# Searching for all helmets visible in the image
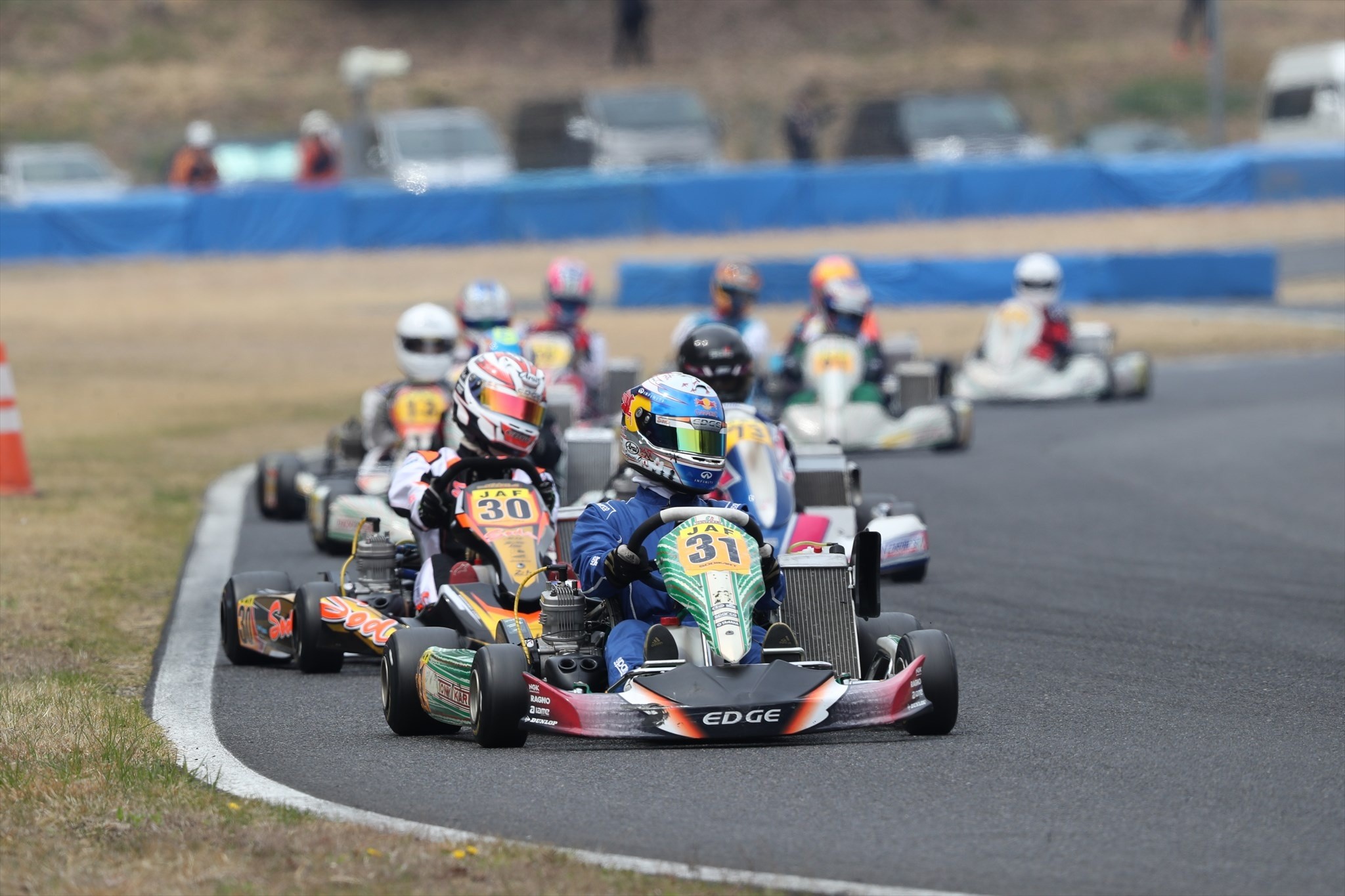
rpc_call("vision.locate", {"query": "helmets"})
[708,259,760,318]
[540,255,594,325]
[807,255,873,338]
[394,303,460,384]
[461,280,513,347]
[1011,251,1062,310]
[451,351,547,455]
[677,325,754,402]
[620,370,729,495]
[799,331,866,397]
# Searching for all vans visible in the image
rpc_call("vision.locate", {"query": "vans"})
[1262,46,1343,149]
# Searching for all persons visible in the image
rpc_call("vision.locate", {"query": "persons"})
[977,251,1070,363]
[569,371,800,693]
[298,109,343,181]
[1175,0,1223,52]
[361,251,887,512]
[169,121,219,188]
[387,351,576,615]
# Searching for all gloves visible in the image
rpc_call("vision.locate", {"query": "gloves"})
[418,485,456,528]
[759,542,781,585]
[532,479,556,513]
[606,543,653,588]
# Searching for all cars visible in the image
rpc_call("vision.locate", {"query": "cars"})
[508,84,727,173]
[787,83,1060,160]
[358,105,517,184]
[3,142,146,201]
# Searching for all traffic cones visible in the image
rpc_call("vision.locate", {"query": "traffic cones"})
[0,338,39,498]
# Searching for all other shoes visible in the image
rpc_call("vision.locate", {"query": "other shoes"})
[549,561,578,582]
[640,624,679,675]
[449,561,479,584]
[760,622,801,663]
